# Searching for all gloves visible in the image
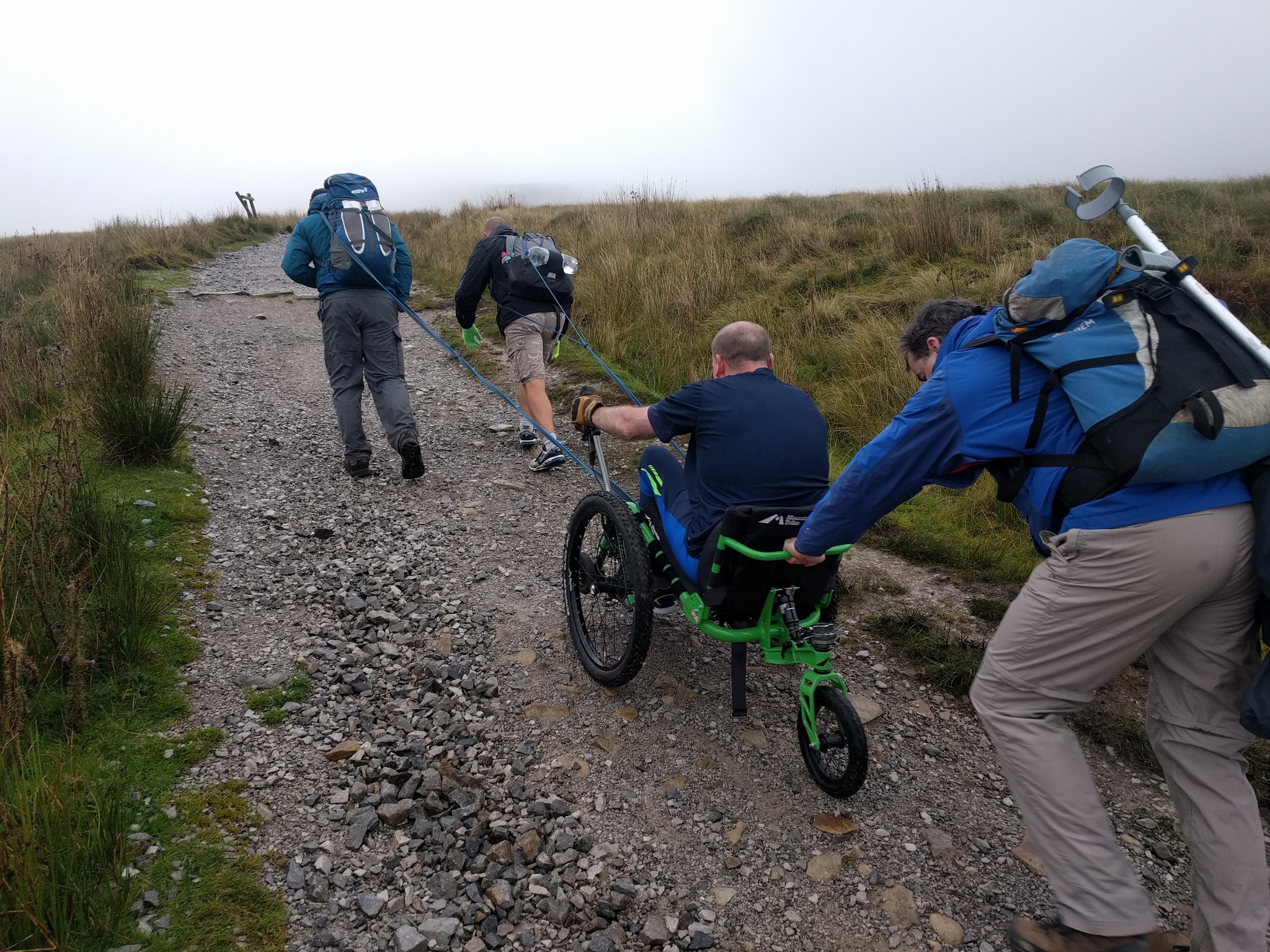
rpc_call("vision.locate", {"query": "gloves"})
[462,324,483,349]
[551,340,560,360]
[570,395,605,434]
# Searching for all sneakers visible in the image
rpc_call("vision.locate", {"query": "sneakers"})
[518,429,539,446]
[529,445,566,472]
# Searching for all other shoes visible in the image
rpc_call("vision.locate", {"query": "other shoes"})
[343,457,370,476]
[652,586,677,615]
[398,429,425,479]
[1012,915,1149,952]
[1160,929,1190,952]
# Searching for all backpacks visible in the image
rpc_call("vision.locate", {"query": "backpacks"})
[958,237,1270,486]
[494,234,574,305]
[323,172,396,286]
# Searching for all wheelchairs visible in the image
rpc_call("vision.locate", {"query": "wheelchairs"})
[554,386,871,799]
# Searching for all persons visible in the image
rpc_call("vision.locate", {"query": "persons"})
[783,301,1270,952]
[281,188,427,478]
[454,217,573,471]
[571,321,831,615]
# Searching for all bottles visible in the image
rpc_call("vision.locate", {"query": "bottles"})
[501,252,520,282]
[523,246,578,275]
